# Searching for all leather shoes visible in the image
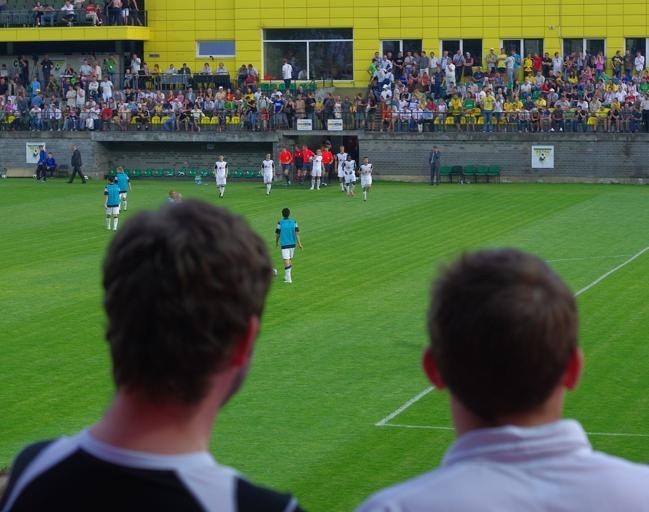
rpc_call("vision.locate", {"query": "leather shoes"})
[66,181,73,183]
[82,180,86,184]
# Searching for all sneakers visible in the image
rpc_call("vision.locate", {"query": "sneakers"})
[317,188,320,190]
[219,196,224,198]
[364,199,366,201]
[322,183,327,186]
[351,194,354,197]
[348,195,350,196]
[113,228,117,231]
[284,280,292,283]
[342,190,346,192]
[310,188,314,190]
[266,194,270,196]
[106,227,110,230]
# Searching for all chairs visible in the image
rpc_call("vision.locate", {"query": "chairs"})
[260,81,318,92]
[463,166,476,183]
[0,0,106,26]
[449,166,462,183]
[105,164,268,179]
[475,165,487,184]
[130,114,244,126]
[432,113,507,127]
[438,166,449,183]
[487,164,501,183]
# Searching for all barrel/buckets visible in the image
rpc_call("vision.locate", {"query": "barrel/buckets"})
[194,175,202,184]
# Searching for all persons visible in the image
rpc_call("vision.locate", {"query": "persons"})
[260,140,373,202]
[351,247,649,512]
[104,177,121,230]
[35,143,56,182]
[68,145,86,184]
[1,53,367,130]
[114,166,131,211]
[275,208,302,283]
[1,197,306,512]
[33,0,143,26]
[367,48,649,133]
[215,154,228,197]
[429,146,440,185]
